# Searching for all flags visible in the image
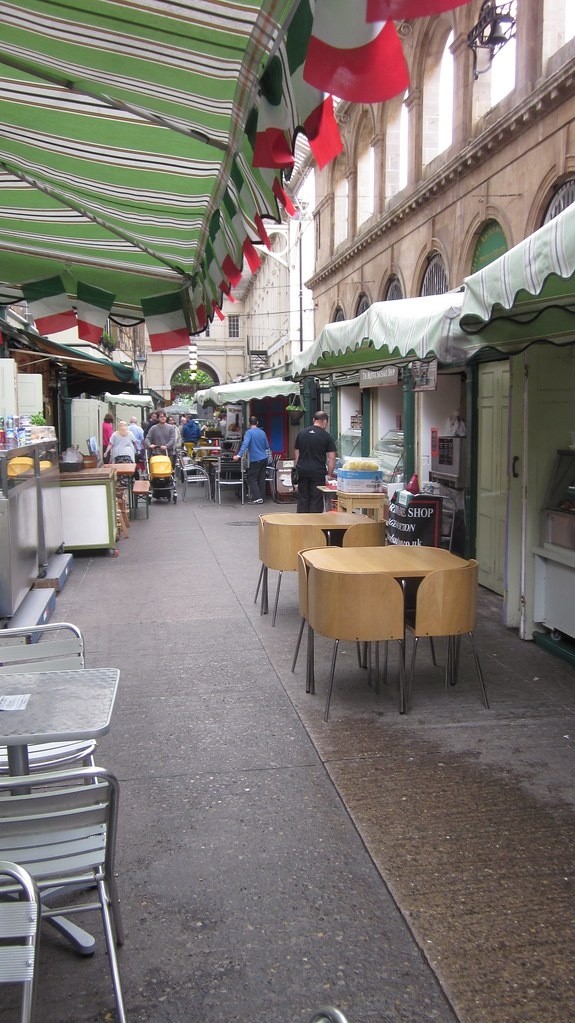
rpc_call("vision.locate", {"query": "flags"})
[19,0,468,350]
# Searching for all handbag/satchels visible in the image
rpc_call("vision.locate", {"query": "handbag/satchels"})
[291,468,299,486]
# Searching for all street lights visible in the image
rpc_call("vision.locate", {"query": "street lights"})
[136,353,147,422]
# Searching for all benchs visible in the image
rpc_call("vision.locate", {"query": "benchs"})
[132,480,151,520]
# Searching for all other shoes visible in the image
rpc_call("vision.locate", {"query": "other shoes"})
[253,498,264,503]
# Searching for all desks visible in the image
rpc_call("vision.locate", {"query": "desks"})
[202,456,235,502]
[194,446,221,457]
[305,546,467,695]
[263,513,380,615]
[104,462,138,519]
[318,486,337,513]
[0,667,122,955]
[338,492,385,523]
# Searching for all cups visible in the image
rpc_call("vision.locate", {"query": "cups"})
[17,428,25,448]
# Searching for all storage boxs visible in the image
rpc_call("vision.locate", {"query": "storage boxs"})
[335,469,383,491]
[83,457,97,469]
[342,456,378,472]
[59,463,81,472]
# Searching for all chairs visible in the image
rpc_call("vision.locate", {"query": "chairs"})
[0,621,128,1023]
[214,457,243,505]
[252,511,490,722]
[178,450,212,502]
[245,453,277,500]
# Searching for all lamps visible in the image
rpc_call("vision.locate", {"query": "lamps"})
[189,351,197,358]
[188,342,197,351]
[188,358,197,365]
[190,370,197,376]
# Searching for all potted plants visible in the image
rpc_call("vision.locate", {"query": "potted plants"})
[100,330,116,352]
[286,406,308,425]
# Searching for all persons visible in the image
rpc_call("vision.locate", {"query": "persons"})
[104,421,139,463]
[141,411,183,477]
[128,416,145,479]
[103,414,113,463]
[232,416,269,504]
[180,415,208,448]
[294,411,336,512]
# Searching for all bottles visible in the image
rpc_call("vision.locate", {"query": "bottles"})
[5,415,15,450]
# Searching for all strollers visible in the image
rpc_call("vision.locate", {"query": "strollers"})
[145,445,177,504]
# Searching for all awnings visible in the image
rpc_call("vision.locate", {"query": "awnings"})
[193,379,301,409]
[292,293,574,378]
[139,389,166,403]
[0,0,301,336]
[7,328,140,384]
[104,393,153,409]
[458,201,574,336]
